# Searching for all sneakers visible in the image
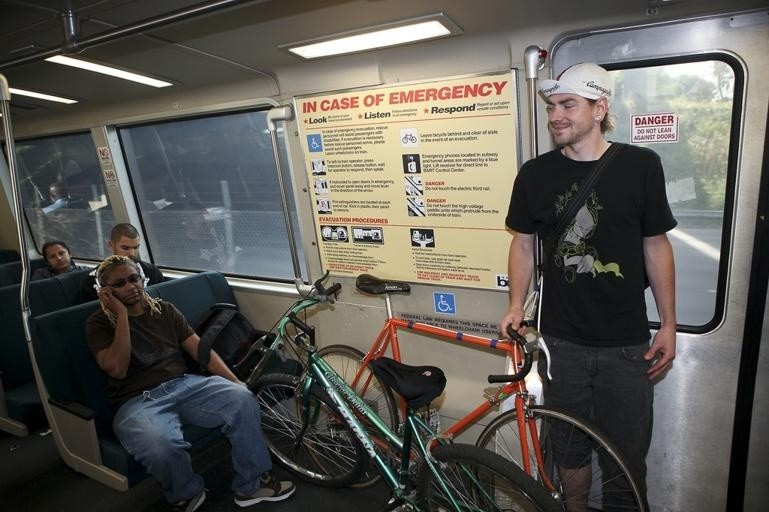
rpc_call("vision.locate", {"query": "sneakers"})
[167,483,208,512]
[231,471,296,508]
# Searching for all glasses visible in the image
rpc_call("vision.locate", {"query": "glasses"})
[98,271,142,290]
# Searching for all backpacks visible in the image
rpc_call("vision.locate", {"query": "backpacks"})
[190,302,266,380]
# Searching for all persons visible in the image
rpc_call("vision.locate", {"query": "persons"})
[85,254,296,511]
[503,62,678,510]
[31,241,81,281]
[77,223,166,304]
[40,182,71,214]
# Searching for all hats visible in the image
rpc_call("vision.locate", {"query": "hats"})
[537,60,615,110]
